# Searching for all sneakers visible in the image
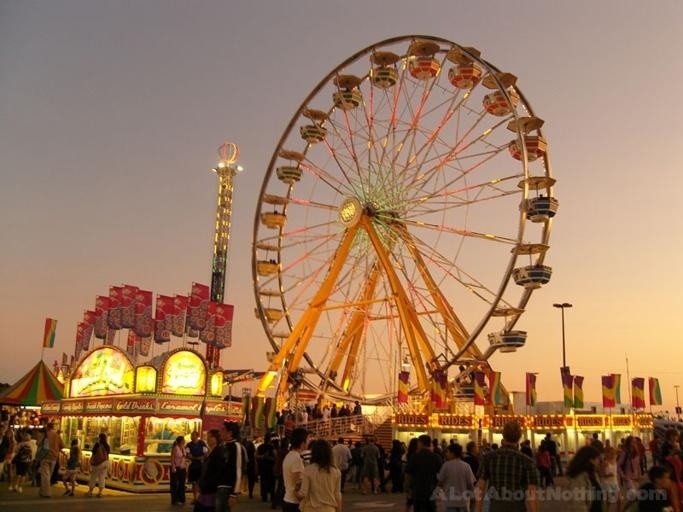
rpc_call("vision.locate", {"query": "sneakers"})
[41,496,51,498]
[96,494,102,497]
[85,493,92,497]
[374,488,381,495]
[69,493,74,496]
[362,490,368,495]
[64,490,70,495]
[176,502,183,505]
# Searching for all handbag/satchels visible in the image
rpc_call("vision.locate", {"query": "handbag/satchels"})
[170,472,183,483]
[90,441,108,466]
[35,432,49,461]
[12,445,26,465]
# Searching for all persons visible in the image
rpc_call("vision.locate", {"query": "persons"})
[1,370,682,512]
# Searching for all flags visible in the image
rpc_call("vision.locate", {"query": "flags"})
[43,281,234,368]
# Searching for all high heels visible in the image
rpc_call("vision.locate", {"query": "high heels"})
[8,485,24,496]
